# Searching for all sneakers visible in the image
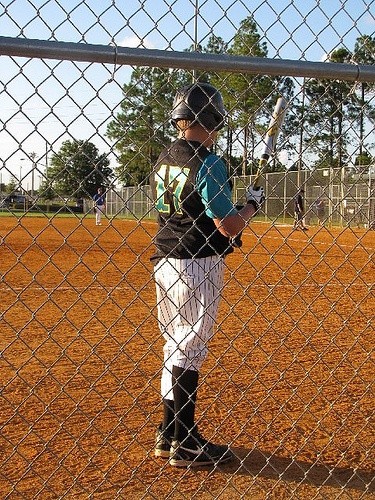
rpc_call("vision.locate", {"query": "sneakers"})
[153,423,174,458]
[292,227,299,231]
[170,438,233,466]
[302,227,309,231]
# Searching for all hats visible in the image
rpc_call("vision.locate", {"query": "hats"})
[299,189,306,193]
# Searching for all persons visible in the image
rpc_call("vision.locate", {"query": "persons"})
[292,189,309,231]
[93,188,106,226]
[315,196,325,226]
[153,83,287,466]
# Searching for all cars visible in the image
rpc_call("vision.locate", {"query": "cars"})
[75,200,83,211]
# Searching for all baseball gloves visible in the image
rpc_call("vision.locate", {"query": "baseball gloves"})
[92,207,96,213]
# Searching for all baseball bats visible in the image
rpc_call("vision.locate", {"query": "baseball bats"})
[230,94,287,245]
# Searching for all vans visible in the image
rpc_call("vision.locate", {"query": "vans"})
[2,195,29,205]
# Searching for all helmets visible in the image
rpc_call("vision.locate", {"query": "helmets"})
[171,82,225,131]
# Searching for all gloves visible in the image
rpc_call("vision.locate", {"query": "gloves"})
[245,183,265,213]
